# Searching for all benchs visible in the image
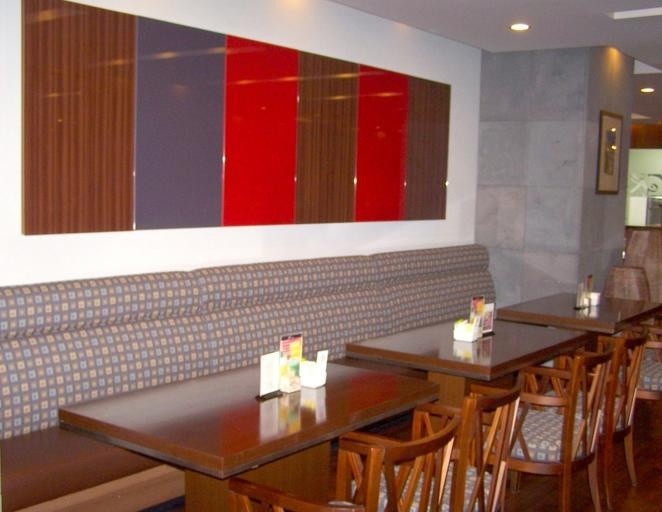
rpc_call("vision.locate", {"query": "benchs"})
[0,245,499,512]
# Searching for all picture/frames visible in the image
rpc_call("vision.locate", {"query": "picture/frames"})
[596,113,624,195]
